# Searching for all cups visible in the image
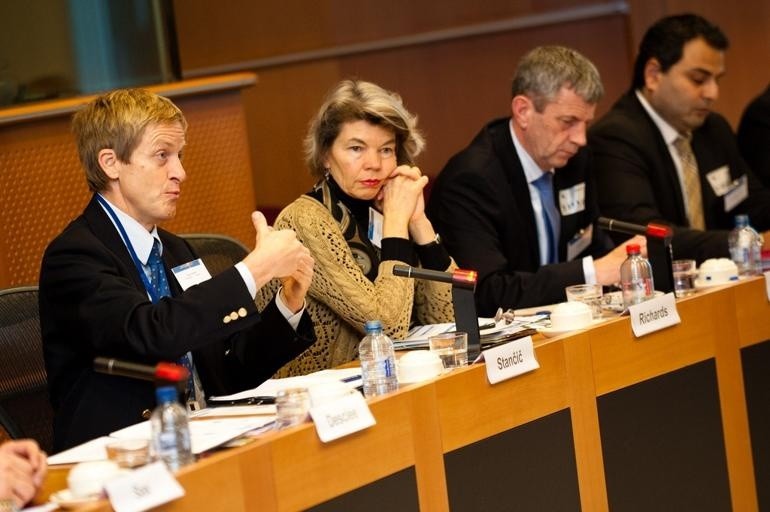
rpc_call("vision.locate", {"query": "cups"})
[68,461,104,495]
[671,259,696,296]
[428,331,469,370]
[551,301,592,328]
[697,258,737,283]
[276,387,311,427]
[567,283,602,321]
[107,440,149,469]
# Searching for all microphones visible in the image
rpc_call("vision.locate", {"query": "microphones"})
[393,264,480,291]
[596,216,675,249]
[92,356,189,392]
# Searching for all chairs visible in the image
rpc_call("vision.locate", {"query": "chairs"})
[1,284,56,456]
[177,231,251,277]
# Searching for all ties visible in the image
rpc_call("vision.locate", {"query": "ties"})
[149,238,195,399]
[532,171,561,265]
[674,131,706,232]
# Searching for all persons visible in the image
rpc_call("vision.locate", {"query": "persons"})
[255,80,460,379]
[586,12,770,269]
[429,45,648,319]
[0,438,48,510]
[737,84,770,187]
[38,89,317,455]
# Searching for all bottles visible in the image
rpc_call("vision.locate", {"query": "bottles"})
[359,321,398,396]
[730,216,762,279]
[153,388,190,467]
[620,244,654,310]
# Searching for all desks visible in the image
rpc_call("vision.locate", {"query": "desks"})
[5,256,764,511]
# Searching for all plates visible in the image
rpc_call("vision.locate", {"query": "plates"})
[48,489,95,509]
[399,350,444,377]
[693,283,732,291]
[396,378,425,387]
[538,329,573,338]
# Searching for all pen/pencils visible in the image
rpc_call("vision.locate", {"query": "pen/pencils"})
[344,375,362,382]
[479,323,495,330]
